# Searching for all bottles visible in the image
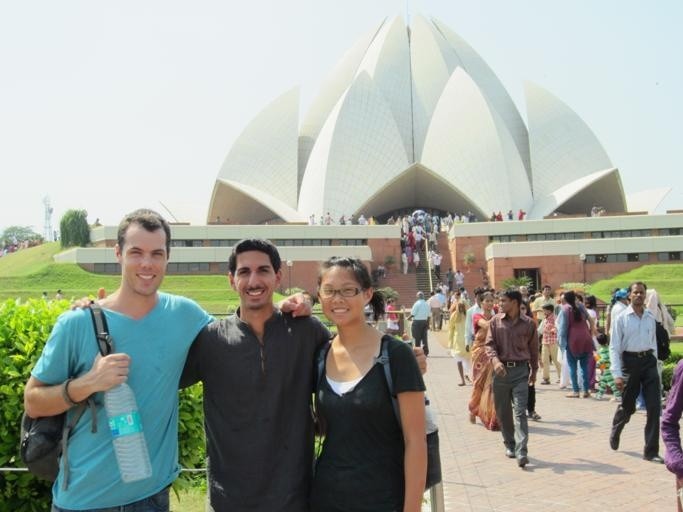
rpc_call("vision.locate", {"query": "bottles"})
[424,397,436,435]
[103,381,152,482]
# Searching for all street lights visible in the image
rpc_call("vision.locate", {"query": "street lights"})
[578,253,589,294]
[286,260,296,294]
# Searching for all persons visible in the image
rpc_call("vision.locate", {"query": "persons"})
[637,288,675,410]
[482,288,539,468]
[364,263,500,430]
[661,358,683,511]
[0,237,42,256]
[213,215,231,225]
[587,205,607,217]
[519,284,629,397]
[24,208,313,512]
[94,217,101,226]
[608,282,664,464]
[310,253,429,511]
[461,209,526,222]
[309,212,376,224]
[66,238,428,512]
[389,213,465,279]
[42,289,64,301]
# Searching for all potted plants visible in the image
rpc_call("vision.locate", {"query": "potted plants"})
[461,253,475,273]
[382,256,397,274]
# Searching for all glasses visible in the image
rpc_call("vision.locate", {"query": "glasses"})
[318,288,362,298]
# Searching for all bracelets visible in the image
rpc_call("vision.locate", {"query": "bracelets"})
[61,378,81,409]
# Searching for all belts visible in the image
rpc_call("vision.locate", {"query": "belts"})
[503,361,527,368]
[623,349,652,358]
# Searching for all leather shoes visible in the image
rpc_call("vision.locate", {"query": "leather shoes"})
[648,456,663,464]
[505,448,528,468]
[609,435,618,450]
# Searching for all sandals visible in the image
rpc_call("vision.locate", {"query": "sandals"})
[528,411,540,419]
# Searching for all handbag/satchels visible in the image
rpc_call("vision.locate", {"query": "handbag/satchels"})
[655,321,669,360]
[20,410,65,481]
